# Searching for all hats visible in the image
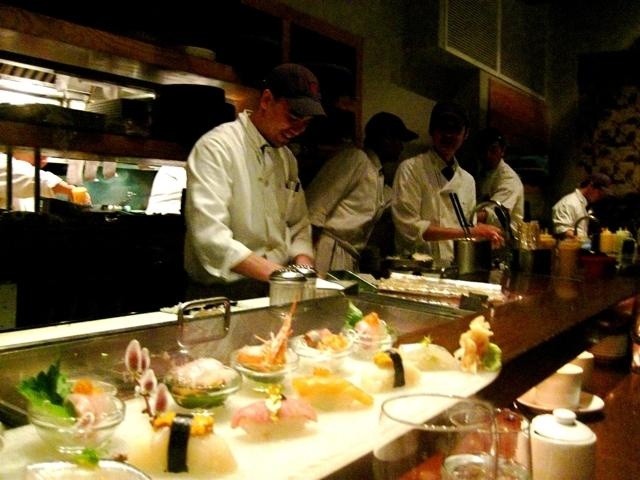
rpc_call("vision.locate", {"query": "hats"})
[430,100,467,125]
[266,63,326,116]
[364,111,419,143]
[587,174,614,196]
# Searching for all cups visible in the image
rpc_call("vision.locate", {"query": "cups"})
[442,408,530,480]
[453,238,492,276]
[571,349,594,384]
[537,363,581,410]
[372,395,499,480]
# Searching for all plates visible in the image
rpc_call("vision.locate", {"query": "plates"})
[1,366,500,480]
[590,335,627,358]
[518,384,604,416]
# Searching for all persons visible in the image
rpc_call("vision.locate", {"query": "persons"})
[475,129,526,232]
[1,149,92,214]
[144,165,188,216]
[305,110,420,281]
[391,96,501,268]
[183,62,315,298]
[551,176,606,239]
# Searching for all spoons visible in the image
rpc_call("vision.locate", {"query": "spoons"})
[494,206,519,250]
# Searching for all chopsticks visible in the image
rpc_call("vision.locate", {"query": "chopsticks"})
[449,192,473,242]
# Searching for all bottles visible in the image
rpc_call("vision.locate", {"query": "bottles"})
[517,409,597,480]
[599,227,631,253]
[267,268,305,320]
[286,263,320,310]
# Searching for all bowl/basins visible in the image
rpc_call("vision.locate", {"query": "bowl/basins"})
[344,325,398,360]
[164,366,246,410]
[229,344,299,394]
[25,395,123,457]
[51,378,117,404]
[21,456,153,480]
[287,330,356,362]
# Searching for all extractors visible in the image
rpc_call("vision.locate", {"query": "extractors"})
[1,62,73,98]
